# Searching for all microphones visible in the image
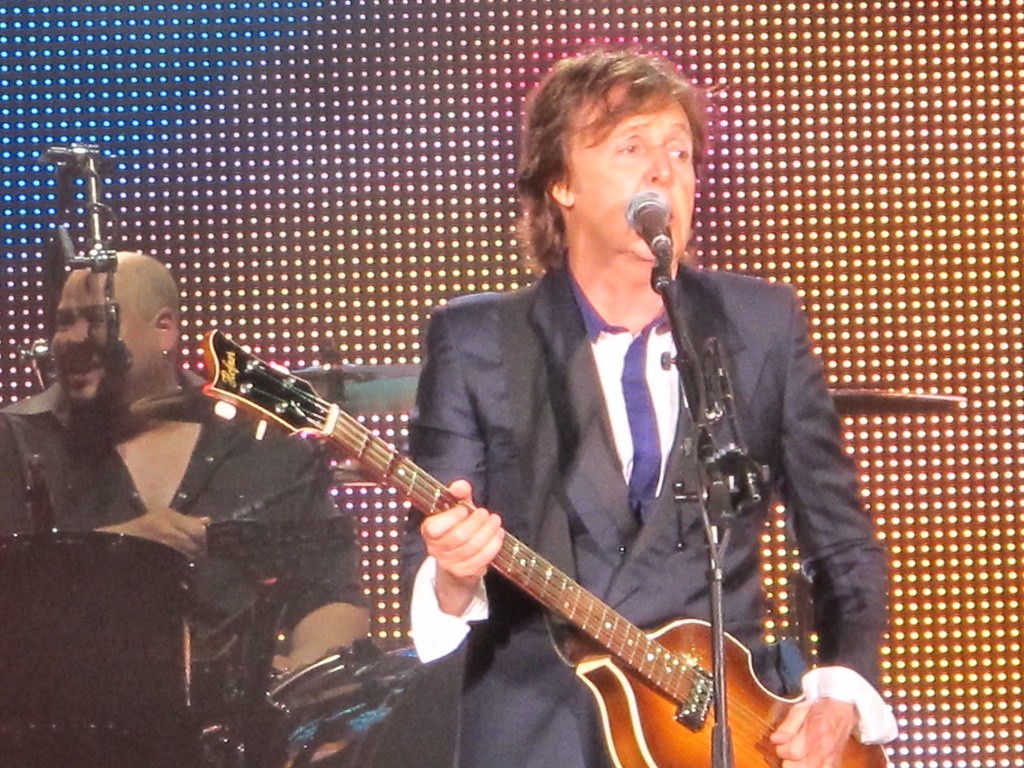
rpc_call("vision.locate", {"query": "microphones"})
[625,191,673,253]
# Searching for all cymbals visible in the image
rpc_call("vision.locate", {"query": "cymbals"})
[128,365,421,421]
[831,387,968,414]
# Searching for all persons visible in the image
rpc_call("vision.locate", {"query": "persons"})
[0,251,371,768]
[399,47,898,768]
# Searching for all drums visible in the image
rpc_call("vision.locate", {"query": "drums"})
[2,531,198,768]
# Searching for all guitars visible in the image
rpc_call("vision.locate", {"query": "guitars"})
[186,316,904,768]
